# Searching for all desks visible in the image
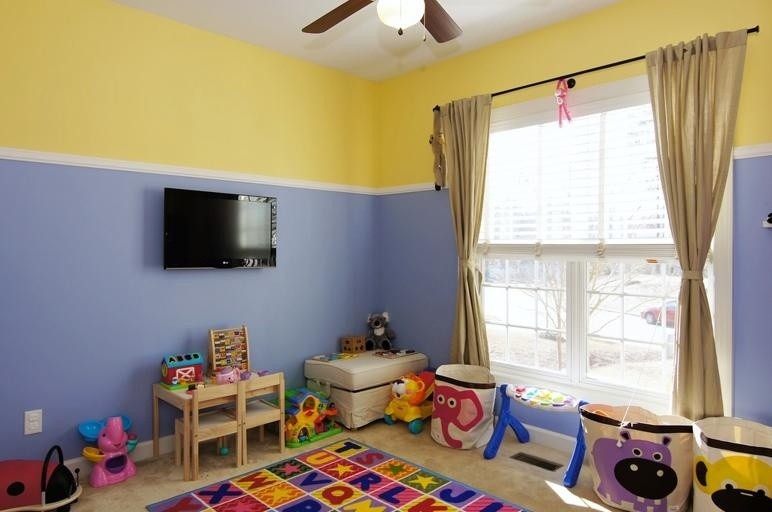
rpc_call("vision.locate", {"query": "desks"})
[151,369,286,482]
[303,347,429,433]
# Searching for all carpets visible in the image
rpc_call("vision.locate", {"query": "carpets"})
[143,436,537,511]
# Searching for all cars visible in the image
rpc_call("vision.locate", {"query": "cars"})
[637,298,678,329]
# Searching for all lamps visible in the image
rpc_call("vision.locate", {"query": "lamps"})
[375,0,430,43]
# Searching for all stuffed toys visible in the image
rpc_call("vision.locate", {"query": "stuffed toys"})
[554,80,572,128]
[366,311,396,352]
[429,106,446,192]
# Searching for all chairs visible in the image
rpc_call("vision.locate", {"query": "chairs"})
[221,370,285,466]
[173,381,244,481]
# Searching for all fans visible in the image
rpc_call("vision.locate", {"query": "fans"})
[300,1,461,46]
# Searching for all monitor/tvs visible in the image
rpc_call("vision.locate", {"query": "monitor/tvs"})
[163,188,276,270]
